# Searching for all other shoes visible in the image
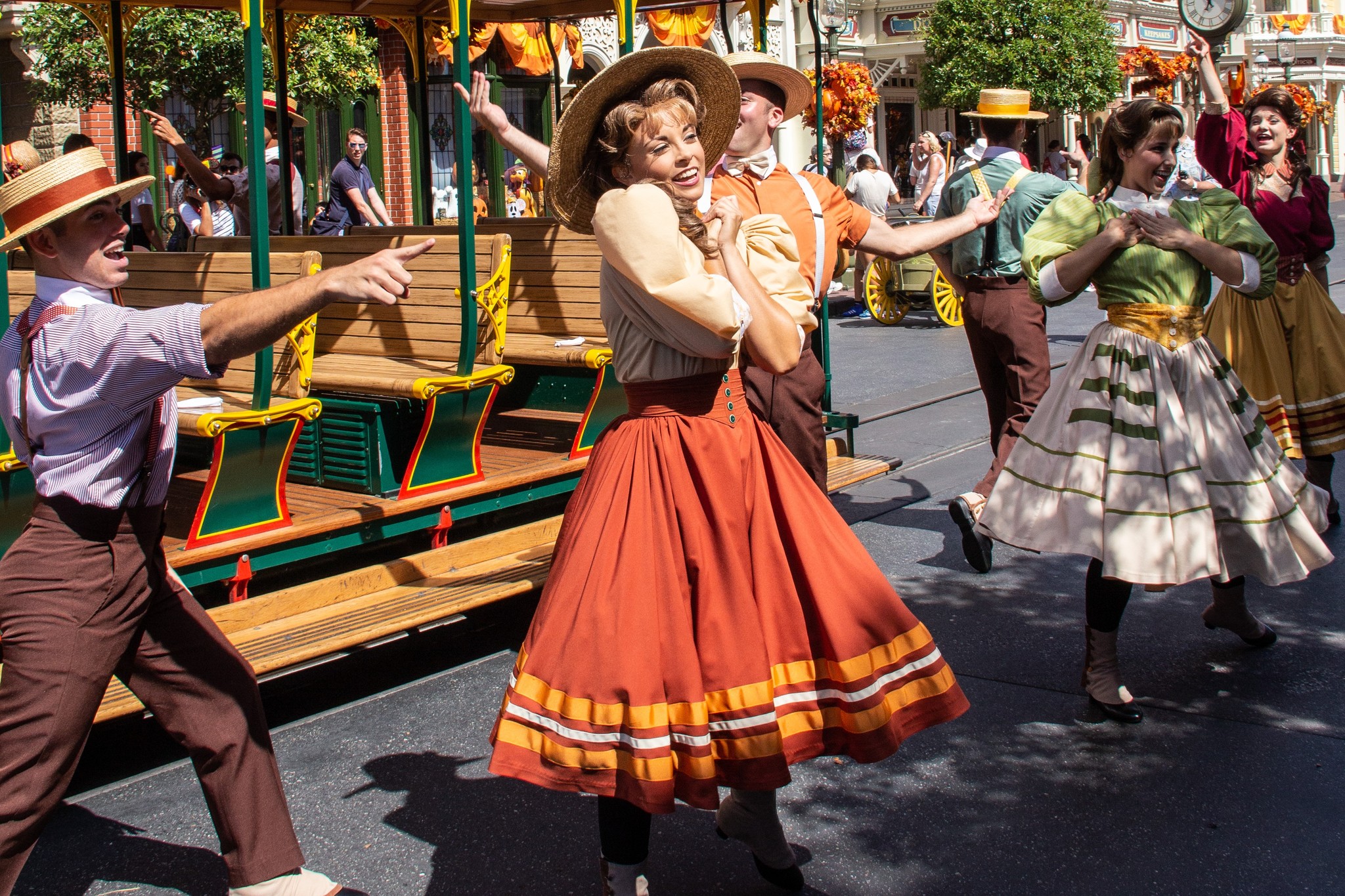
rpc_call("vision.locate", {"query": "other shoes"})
[949,492,994,573]
[226,872,369,896]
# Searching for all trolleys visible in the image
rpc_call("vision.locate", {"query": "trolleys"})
[862,208,964,327]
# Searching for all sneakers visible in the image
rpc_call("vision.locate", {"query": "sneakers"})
[842,306,864,317]
[859,308,885,319]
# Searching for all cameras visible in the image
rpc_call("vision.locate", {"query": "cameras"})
[184,174,199,189]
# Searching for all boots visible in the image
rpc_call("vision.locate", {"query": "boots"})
[716,790,805,891]
[1201,574,1277,647]
[1082,621,1143,724]
[598,848,672,896]
[1303,455,1341,526]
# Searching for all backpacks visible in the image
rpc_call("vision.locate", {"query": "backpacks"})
[1043,152,1060,175]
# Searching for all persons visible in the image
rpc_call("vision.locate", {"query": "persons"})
[1161,104,1222,202]
[143,90,310,236]
[167,149,244,252]
[1184,28,1345,525]
[843,115,884,184]
[921,88,1088,574]
[327,128,398,236]
[452,46,1009,504]
[1077,133,1095,178]
[801,143,843,294]
[840,154,901,319]
[125,151,166,252]
[909,129,988,218]
[973,96,1334,723]
[1043,140,1068,181]
[1,140,436,894]
[488,49,974,896]
[1,133,95,182]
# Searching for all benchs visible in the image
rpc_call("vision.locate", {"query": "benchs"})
[0,218,629,552]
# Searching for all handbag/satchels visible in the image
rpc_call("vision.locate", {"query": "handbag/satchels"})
[309,219,345,236]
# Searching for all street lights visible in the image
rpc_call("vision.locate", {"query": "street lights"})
[808,0,858,288]
[1271,23,1304,86]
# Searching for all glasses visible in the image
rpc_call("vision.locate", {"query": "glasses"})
[822,152,833,157]
[219,165,241,173]
[346,142,366,148]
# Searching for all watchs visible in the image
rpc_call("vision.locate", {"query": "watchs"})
[1191,181,1198,191]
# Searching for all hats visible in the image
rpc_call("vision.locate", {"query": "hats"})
[939,132,957,140]
[1,140,42,182]
[235,91,309,127]
[963,138,987,161]
[0,146,156,253]
[543,45,742,236]
[960,89,1050,120]
[721,51,813,123]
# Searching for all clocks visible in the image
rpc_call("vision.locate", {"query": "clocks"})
[1178,0,1248,36]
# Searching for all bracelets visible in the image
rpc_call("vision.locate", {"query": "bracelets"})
[387,221,394,226]
